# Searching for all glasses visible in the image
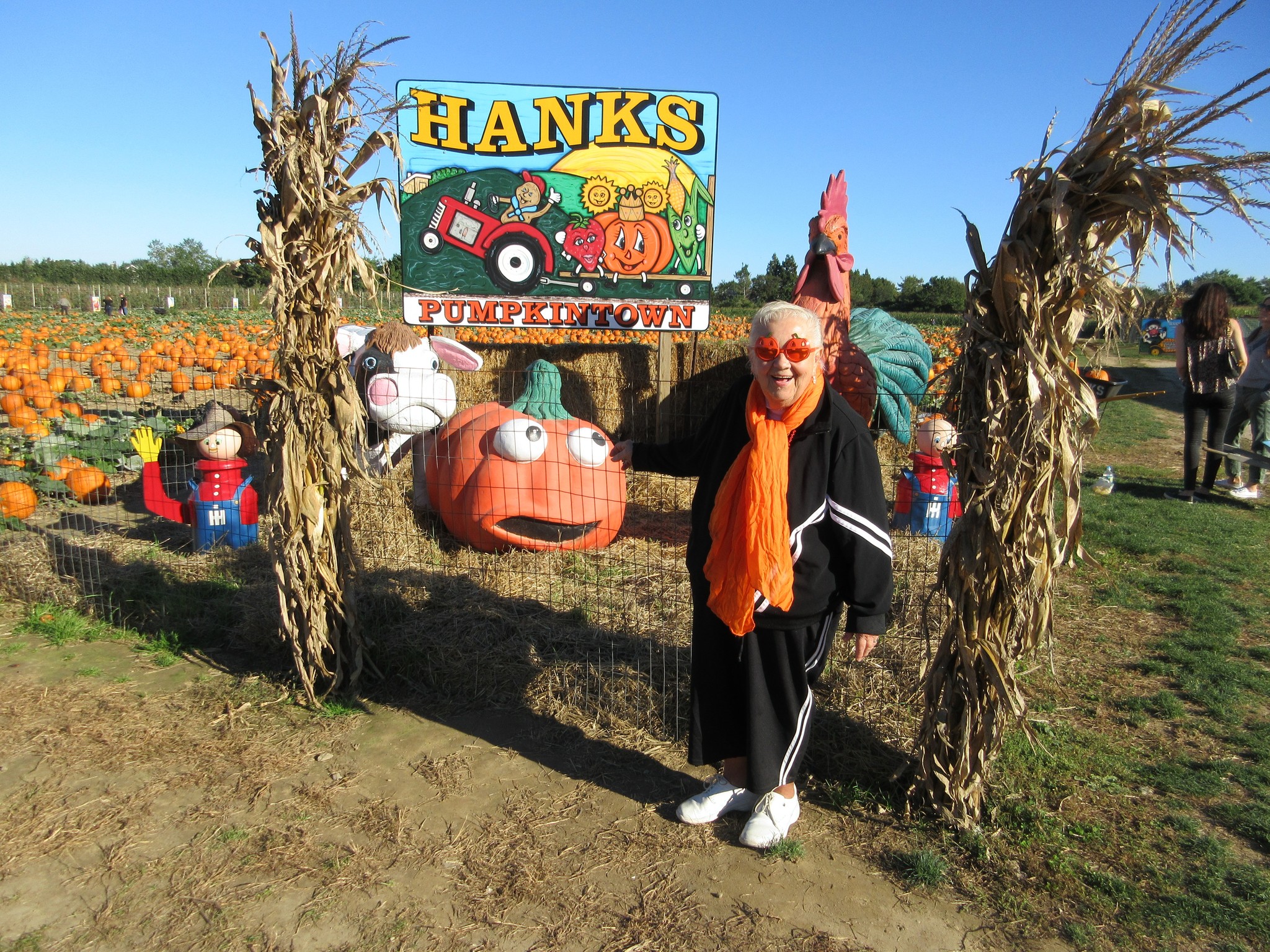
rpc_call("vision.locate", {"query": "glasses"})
[1259,303,1270,311]
[749,334,819,363]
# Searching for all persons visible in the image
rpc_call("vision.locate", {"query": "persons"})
[1163,283,1248,503]
[610,299,895,850]
[57,295,71,316]
[119,293,128,315]
[103,297,113,317]
[1208,296,1270,499]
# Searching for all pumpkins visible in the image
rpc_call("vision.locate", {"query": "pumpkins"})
[0,482,37,518]
[1086,369,1110,382]
[66,467,110,503]
[339,305,751,345]
[917,324,962,424]
[0,306,283,481]
[1068,362,1081,376]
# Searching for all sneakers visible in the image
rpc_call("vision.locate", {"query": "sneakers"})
[1179,489,1210,502]
[737,779,800,848]
[676,773,759,825]
[1226,487,1258,498]
[1214,479,1243,489]
[1163,493,1193,505]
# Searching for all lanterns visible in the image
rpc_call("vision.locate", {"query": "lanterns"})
[424,360,626,553]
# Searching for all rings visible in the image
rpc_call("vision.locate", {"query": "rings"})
[873,640,877,642]
